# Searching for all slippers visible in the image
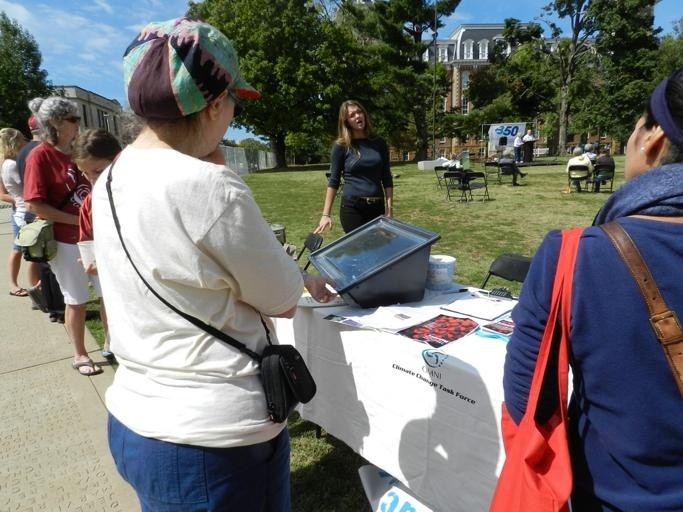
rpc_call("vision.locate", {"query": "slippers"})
[102,351,119,364]
[72,358,103,376]
[9,287,29,296]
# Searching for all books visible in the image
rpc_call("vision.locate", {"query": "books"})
[482,313,515,336]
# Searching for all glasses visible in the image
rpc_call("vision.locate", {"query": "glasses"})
[79,166,104,179]
[60,115,82,124]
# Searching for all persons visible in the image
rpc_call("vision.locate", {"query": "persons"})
[495,135,528,186]
[522,129,539,142]
[16,115,44,288]
[591,148,616,192]
[513,133,524,164]
[565,146,594,193]
[313,99,393,234]
[73,127,122,276]
[583,143,597,166]
[1,128,40,297]
[22,96,115,376]
[502,75,683,512]
[91,18,338,512]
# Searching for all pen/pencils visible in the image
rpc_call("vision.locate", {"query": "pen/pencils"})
[442,288,468,294]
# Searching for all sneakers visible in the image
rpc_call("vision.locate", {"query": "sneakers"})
[31,300,40,310]
[29,287,49,312]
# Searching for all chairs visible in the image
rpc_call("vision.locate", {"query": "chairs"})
[567,165,592,193]
[443,172,467,201]
[484,160,503,184]
[448,167,475,189]
[479,252,532,289]
[434,166,455,190]
[499,163,517,186]
[463,171,489,203]
[592,164,616,193]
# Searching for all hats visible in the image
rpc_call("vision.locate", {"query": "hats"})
[122,17,260,121]
[584,143,594,151]
[29,114,39,132]
[573,147,582,155]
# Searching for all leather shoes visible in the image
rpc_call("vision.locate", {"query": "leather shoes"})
[521,172,529,178]
[56,315,66,323]
[49,313,57,322]
[513,182,519,186]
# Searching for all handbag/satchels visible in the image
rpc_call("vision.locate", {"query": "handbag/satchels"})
[105,149,317,424]
[14,174,84,263]
[487,225,586,511]
[598,223,682,393]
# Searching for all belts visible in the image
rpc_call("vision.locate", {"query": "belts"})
[347,197,384,206]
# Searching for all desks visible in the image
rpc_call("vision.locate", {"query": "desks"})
[269,280,575,512]
[523,141,535,163]
